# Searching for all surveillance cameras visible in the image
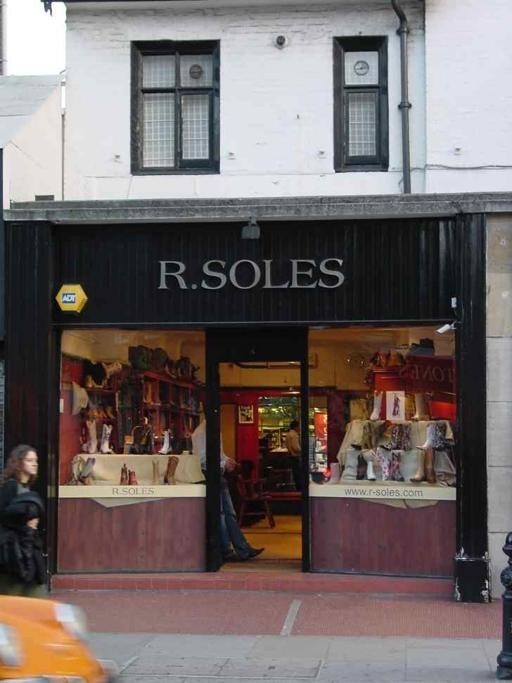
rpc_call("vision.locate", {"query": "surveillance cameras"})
[435,324,451,336]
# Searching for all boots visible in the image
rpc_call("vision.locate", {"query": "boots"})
[164,456,179,484]
[329,462,342,483]
[411,338,434,354]
[71,457,95,485]
[81,376,200,454]
[370,390,384,420]
[120,465,137,485]
[151,460,160,485]
[411,393,430,421]
[351,420,455,484]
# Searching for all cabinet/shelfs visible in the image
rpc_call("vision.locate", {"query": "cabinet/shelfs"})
[81,368,200,453]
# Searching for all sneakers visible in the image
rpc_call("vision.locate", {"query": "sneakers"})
[225,551,240,562]
[243,548,264,560]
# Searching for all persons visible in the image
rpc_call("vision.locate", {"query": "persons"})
[392,393,400,416]
[0,444,52,598]
[285,420,305,516]
[258,433,278,483]
[191,401,265,563]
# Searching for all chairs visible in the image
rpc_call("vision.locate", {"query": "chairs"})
[234,465,303,529]
